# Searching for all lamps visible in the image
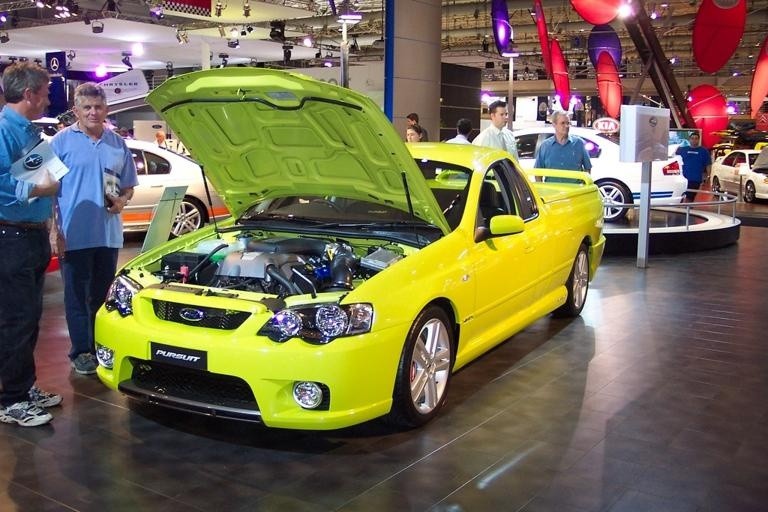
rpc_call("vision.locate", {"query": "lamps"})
[1,33,10,44]
[105,1,117,13]
[92,20,105,33]
[122,57,133,68]
[35,58,42,66]
[19,57,28,63]
[46,2,54,8]
[37,1,44,8]
[8,55,17,62]
[83,16,91,26]
[147,1,361,78]
[0,11,9,23]
[53,1,79,19]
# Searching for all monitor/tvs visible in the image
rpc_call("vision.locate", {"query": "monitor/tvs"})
[667,129,700,157]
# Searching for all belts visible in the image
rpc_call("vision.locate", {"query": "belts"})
[0,221,47,230]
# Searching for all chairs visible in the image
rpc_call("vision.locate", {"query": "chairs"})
[447,183,504,229]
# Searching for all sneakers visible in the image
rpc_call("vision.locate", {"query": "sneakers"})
[70,353,98,374]
[0,401,53,427]
[29,386,62,407]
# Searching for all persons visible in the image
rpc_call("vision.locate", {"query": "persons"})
[445,118,473,145]
[675,132,712,209]
[56,121,67,131]
[471,99,519,164]
[0,61,64,429]
[533,109,592,184]
[406,124,423,142]
[153,131,170,150]
[50,81,140,377]
[119,126,134,139]
[406,112,429,142]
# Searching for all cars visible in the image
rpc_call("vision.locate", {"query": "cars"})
[710,149,768,202]
[94,67,606,433]
[114,137,233,239]
[510,126,690,222]
